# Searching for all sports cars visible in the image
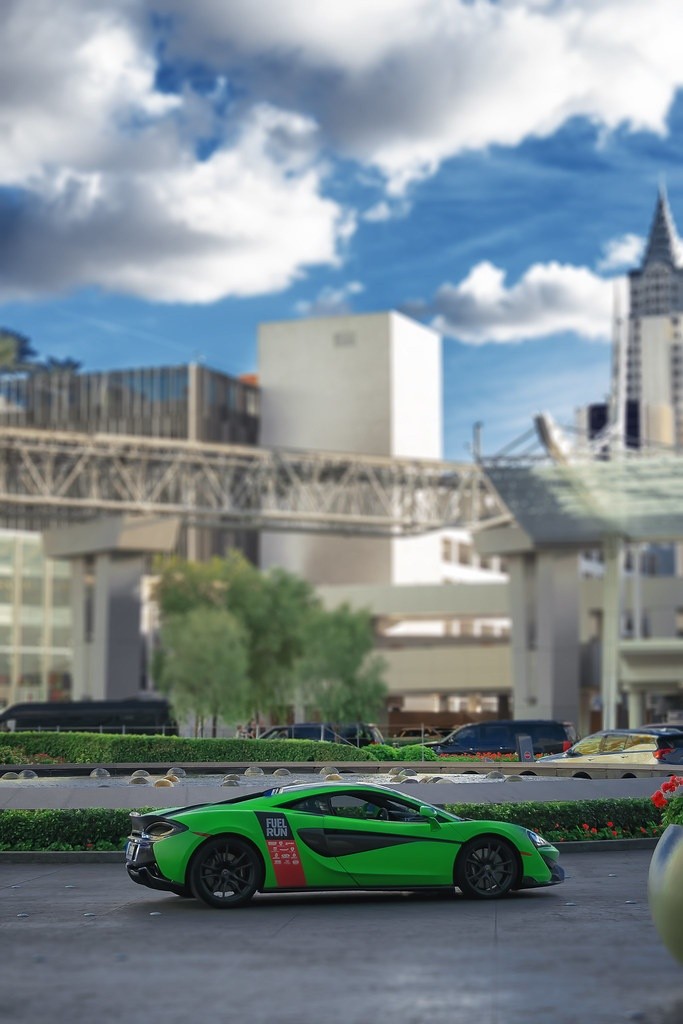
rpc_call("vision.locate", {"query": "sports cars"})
[124,781,566,909]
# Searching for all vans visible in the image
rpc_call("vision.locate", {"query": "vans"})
[0,695,179,736]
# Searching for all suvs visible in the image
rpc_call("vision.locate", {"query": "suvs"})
[416,720,581,755]
[537,726,683,765]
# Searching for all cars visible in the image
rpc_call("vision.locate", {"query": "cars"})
[394,728,441,736]
[258,723,385,749]
